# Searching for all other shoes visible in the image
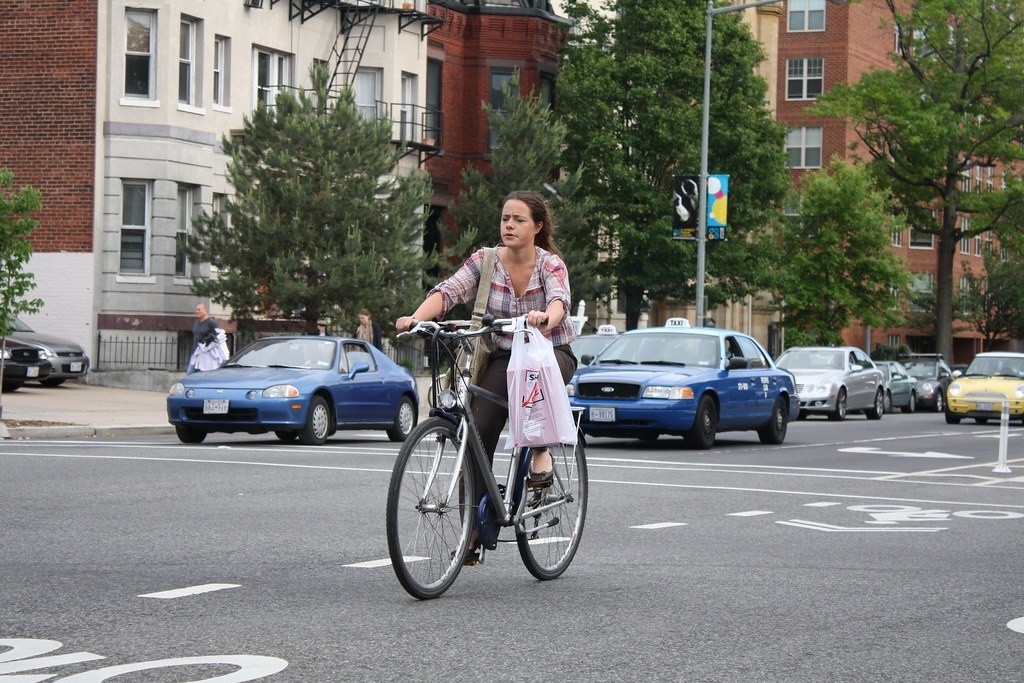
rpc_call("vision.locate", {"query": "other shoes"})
[531,453,554,487]
[451,537,480,561]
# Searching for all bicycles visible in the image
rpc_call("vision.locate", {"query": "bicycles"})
[385,316,590,600]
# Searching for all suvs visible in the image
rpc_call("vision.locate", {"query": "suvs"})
[891,352,956,413]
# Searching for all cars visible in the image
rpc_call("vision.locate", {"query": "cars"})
[558,323,623,375]
[565,318,801,449]
[944,352,1024,423]
[0,335,53,394]
[775,346,886,421]
[874,361,919,414]
[3,312,90,386]
[948,364,968,380]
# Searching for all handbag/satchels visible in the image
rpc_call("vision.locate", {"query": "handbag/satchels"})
[444,316,493,410]
[506,315,577,448]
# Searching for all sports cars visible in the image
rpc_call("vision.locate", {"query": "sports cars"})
[166,335,420,445]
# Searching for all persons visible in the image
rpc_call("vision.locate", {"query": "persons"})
[692,340,729,369]
[394,190,579,561]
[316,317,329,336]
[355,309,383,352]
[187,303,230,374]
